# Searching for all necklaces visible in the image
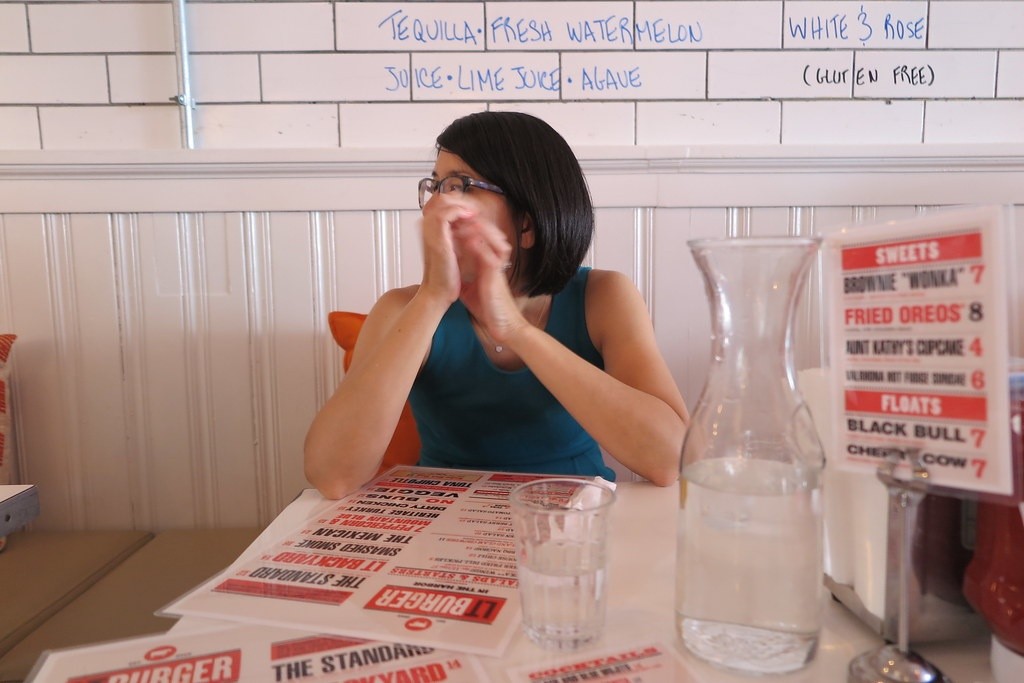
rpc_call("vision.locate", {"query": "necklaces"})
[472,296,548,354]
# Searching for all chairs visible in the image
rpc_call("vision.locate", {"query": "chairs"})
[327,311,421,471]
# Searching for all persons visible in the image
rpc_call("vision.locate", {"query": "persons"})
[300,109,704,500]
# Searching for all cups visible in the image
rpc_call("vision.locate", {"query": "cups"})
[508,479,617,651]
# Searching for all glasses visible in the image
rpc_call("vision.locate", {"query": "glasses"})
[418,176,506,208]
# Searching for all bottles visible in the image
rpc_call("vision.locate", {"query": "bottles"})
[678,232,828,676]
[961,402,1024,683]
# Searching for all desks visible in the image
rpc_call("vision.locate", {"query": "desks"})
[0,530,261,683]
[0,530,154,658]
